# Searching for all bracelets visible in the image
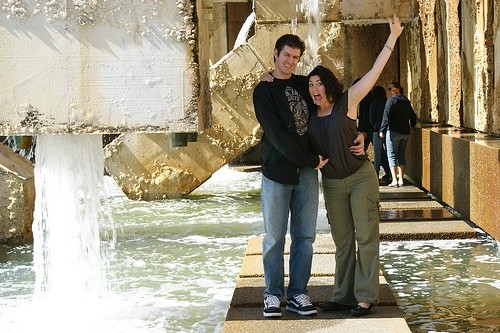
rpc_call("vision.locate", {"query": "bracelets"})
[384,44,395,51]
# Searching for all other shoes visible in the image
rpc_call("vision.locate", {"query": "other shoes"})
[382,174,392,180]
[398,182,403,186]
[351,303,374,316]
[388,183,398,187]
[318,302,354,311]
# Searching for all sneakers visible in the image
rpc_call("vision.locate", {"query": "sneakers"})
[285,294,318,316]
[263,293,283,317]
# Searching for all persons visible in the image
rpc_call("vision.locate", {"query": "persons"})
[385,87,392,98]
[352,78,373,151]
[378,82,417,187]
[261,13,407,316]
[252,34,331,318]
[369,86,392,179]
